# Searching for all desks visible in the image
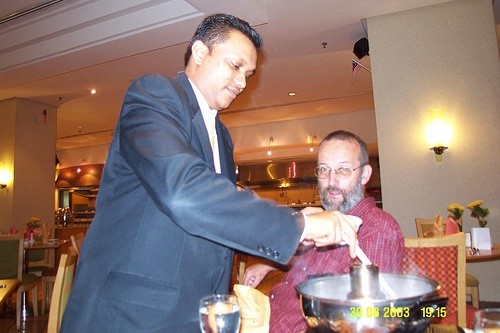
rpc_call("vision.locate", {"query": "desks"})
[20,239,72,323]
[466,244,500,263]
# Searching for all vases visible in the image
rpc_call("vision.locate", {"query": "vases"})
[470,227,493,251]
[29,233,35,246]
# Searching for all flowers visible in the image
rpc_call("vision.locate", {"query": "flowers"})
[467,200,491,228]
[447,202,465,232]
[25,217,42,233]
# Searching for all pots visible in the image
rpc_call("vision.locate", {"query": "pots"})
[294,272,448,333]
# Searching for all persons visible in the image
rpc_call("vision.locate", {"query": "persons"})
[56,13,363,333]
[240,129,405,333]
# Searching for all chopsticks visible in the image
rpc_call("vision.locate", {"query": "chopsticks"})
[357,245,398,300]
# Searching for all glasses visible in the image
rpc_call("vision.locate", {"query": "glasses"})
[313,163,367,179]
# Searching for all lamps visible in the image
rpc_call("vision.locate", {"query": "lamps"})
[428,144,449,162]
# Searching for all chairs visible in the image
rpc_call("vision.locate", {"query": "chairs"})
[41,232,85,316]
[22,227,52,315]
[47,247,78,333]
[402,231,468,333]
[415,218,480,309]
[0,228,39,330]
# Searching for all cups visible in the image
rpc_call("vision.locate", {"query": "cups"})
[474,308,500,333]
[199,294,241,333]
